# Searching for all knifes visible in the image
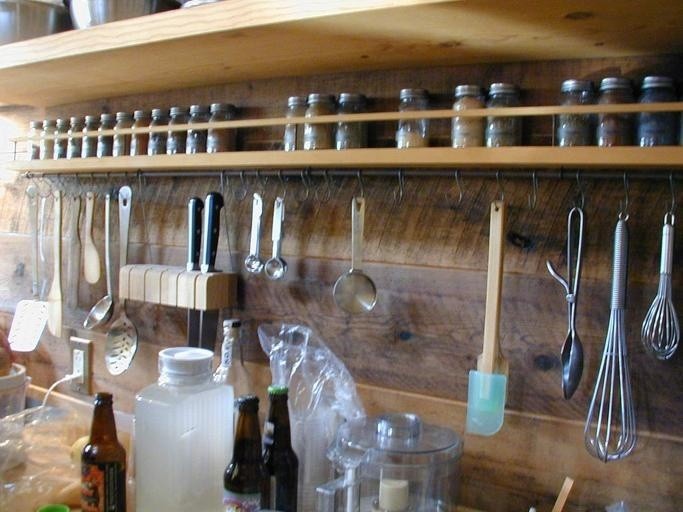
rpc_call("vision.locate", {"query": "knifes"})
[185,191,226,352]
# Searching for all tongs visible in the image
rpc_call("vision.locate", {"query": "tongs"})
[545,208,586,404]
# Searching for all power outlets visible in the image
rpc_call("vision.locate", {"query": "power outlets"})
[66,336,94,398]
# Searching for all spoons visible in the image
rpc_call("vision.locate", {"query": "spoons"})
[243,193,289,281]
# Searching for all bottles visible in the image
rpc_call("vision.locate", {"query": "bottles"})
[557,75,677,147]
[133,319,299,512]
[282,91,368,151]
[450,82,524,148]
[81,391,126,511]
[27,102,235,160]
[396,87,429,149]
